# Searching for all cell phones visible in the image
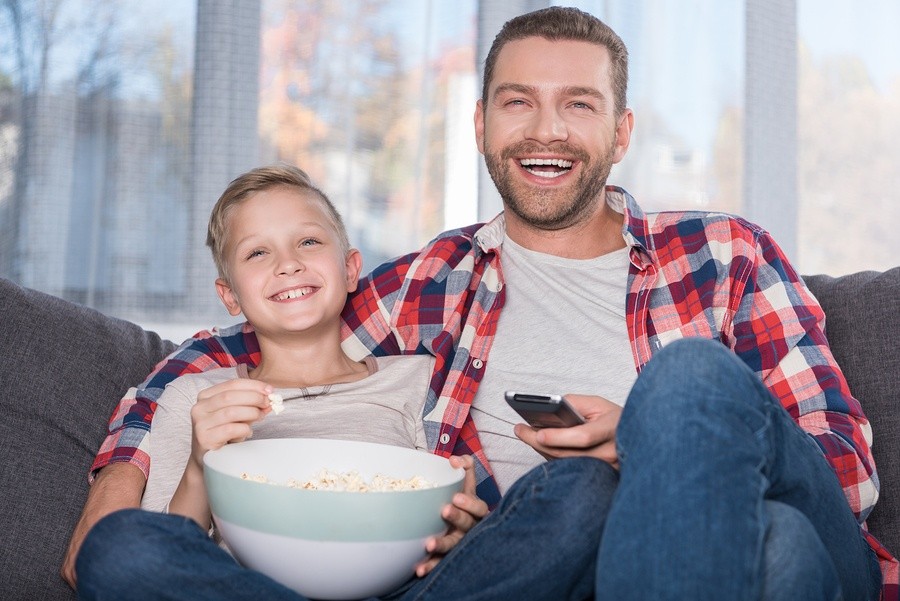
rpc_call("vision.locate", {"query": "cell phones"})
[503,390,590,429]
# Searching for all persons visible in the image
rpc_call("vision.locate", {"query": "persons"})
[72,160,622,601]
[59,6,900,601]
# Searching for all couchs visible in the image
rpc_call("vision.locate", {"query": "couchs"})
[0,266,900,601]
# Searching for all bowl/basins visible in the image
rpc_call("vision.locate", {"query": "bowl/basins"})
[201,438,467,600]
